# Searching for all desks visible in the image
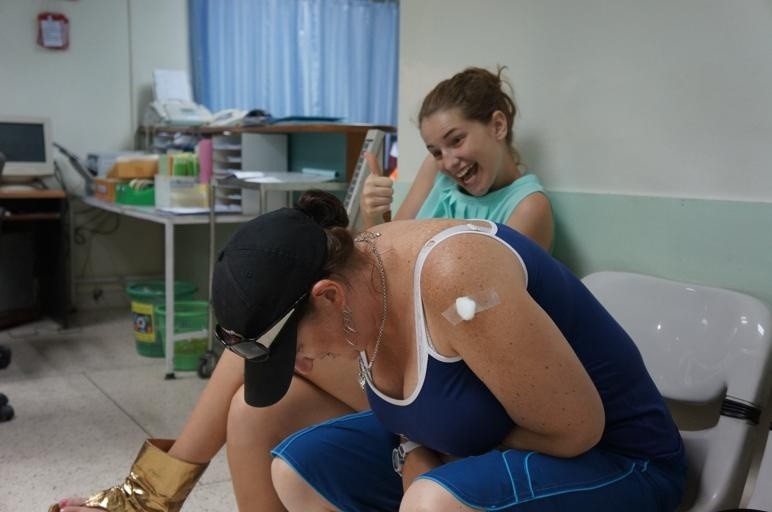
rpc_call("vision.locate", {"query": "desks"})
[199,177,351,379]
[71,190,242,379]
[0,187,68,329]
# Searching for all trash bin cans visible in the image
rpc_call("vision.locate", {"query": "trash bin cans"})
[154,301,209,371]
[127,282,199,357]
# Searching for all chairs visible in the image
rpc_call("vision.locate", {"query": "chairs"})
[744,416,772,512]
[579,270,772,512]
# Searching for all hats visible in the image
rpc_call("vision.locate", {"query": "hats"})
[214,207,328,407]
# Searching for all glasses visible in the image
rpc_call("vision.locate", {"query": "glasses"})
[214,291,310,362]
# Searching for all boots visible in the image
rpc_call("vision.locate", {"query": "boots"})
[48,438,210,512]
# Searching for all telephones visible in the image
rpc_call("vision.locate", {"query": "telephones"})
[150,98,212,125]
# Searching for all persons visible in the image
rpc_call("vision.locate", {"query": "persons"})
[211,188,697,511]
[50,67,556,512]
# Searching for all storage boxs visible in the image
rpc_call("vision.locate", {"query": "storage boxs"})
[153,173,199,211]
[109,159,158,180]
[94,178,119,203]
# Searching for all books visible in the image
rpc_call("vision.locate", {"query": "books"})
[215,166,341,184]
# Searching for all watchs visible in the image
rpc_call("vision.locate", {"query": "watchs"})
[392,441,423,477]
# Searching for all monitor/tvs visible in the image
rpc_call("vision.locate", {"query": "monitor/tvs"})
[0,116,55,192]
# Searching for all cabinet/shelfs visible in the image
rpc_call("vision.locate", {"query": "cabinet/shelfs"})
[210,133,290,215]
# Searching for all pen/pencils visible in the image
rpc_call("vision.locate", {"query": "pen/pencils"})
[168,150,200,177]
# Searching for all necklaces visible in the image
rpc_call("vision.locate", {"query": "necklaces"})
[354,231,387,392]
